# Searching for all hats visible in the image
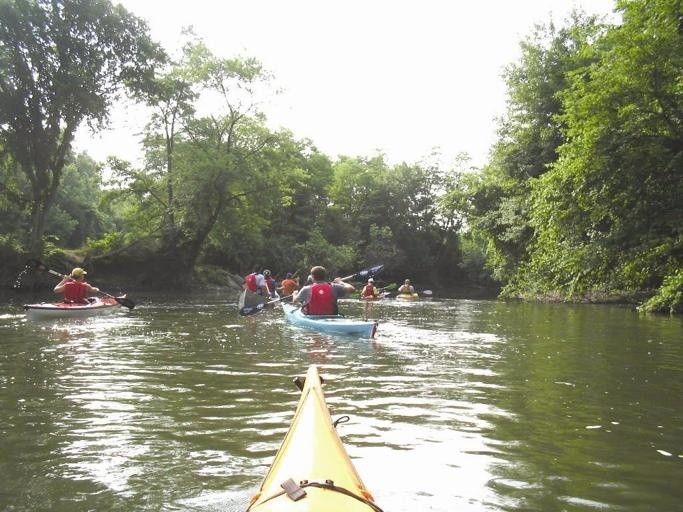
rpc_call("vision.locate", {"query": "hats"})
[72,268,88,277]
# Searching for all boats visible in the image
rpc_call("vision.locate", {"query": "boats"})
[278,301,378,340]
[358,295,383,301]
[21,293,128,318]
[246,361,383,512]
[238,288,281,312]
[395,292,420,302]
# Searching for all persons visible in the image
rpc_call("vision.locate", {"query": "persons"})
[290,266,356,315]
[360,278,382,298]
[52,268,99,303]
[397,277,415,295]
[244,264,313,299]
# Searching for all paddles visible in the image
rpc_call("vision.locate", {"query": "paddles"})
[352,282,397,298]
[26,259,135,309]
[383,290,432,295]
[239,264,384,315]
